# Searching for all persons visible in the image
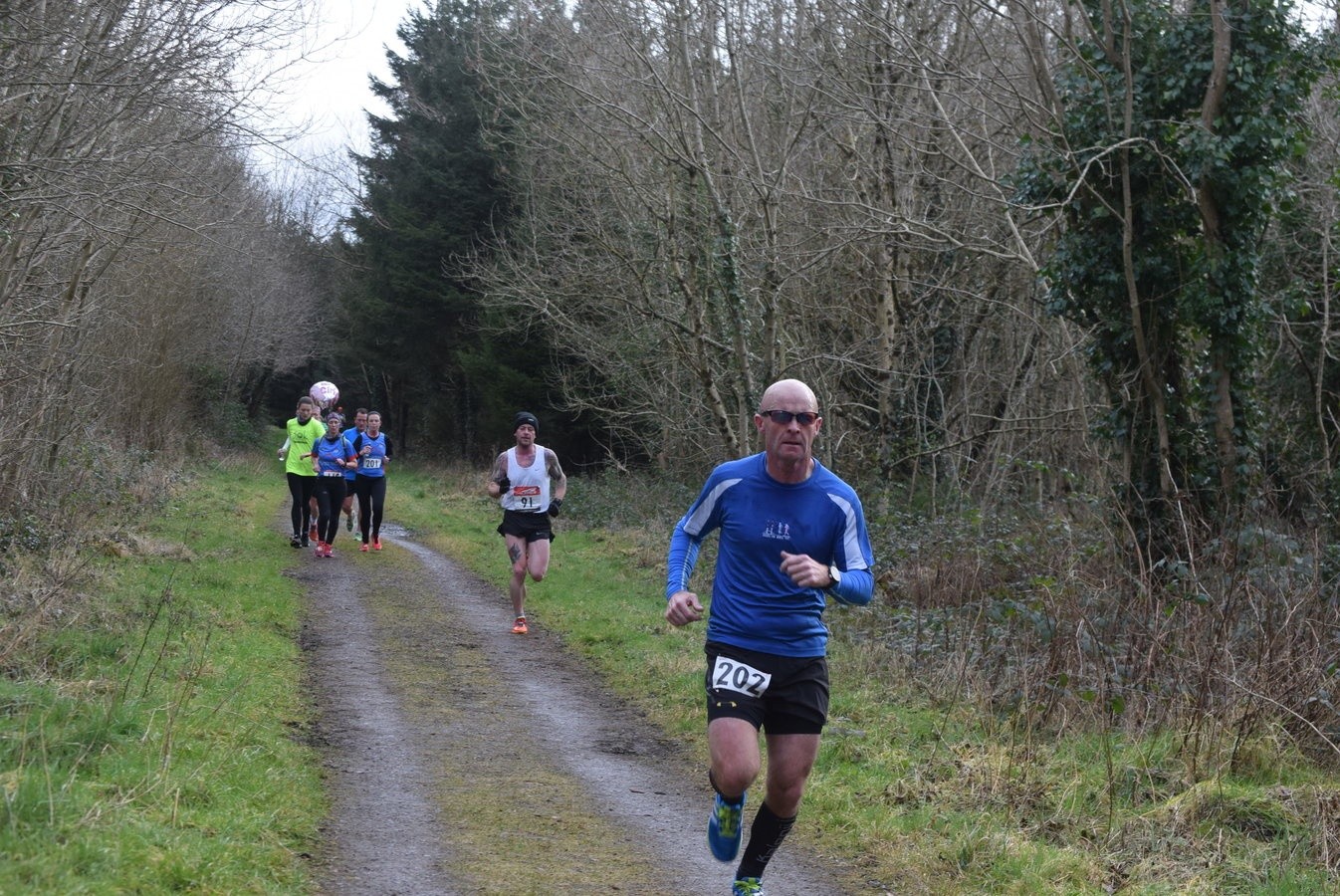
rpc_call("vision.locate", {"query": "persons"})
[278,397,394,558]
[488,412,566,633]
[665,378,877,896]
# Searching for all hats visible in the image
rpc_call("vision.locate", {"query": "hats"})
[512,411,539,438]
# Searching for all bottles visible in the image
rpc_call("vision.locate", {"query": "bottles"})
[279,449,285,461]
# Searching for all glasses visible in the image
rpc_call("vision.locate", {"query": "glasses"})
[760,410,818,426]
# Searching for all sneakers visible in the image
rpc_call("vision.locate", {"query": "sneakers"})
[325,543,333,557]
[360,542,370,551]
[314,541,325,557]
[301,528,310,547]
[354,532,362,541]
[347,510,356,532]
[371,532,382,550]
[291,535,302,548]
[309,523,318,541]
[732,873,765,896]
[706,791,746,862]
[512,617,527,634]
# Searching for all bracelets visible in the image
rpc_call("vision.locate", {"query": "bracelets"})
[343,462,347,467]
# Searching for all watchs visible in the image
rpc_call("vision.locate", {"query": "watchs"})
[822,563,841,590]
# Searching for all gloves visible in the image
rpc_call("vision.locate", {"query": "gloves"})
[499,476,511,493]
[547,497,562,517]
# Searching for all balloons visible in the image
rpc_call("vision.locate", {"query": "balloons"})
[309,381,339,410]
